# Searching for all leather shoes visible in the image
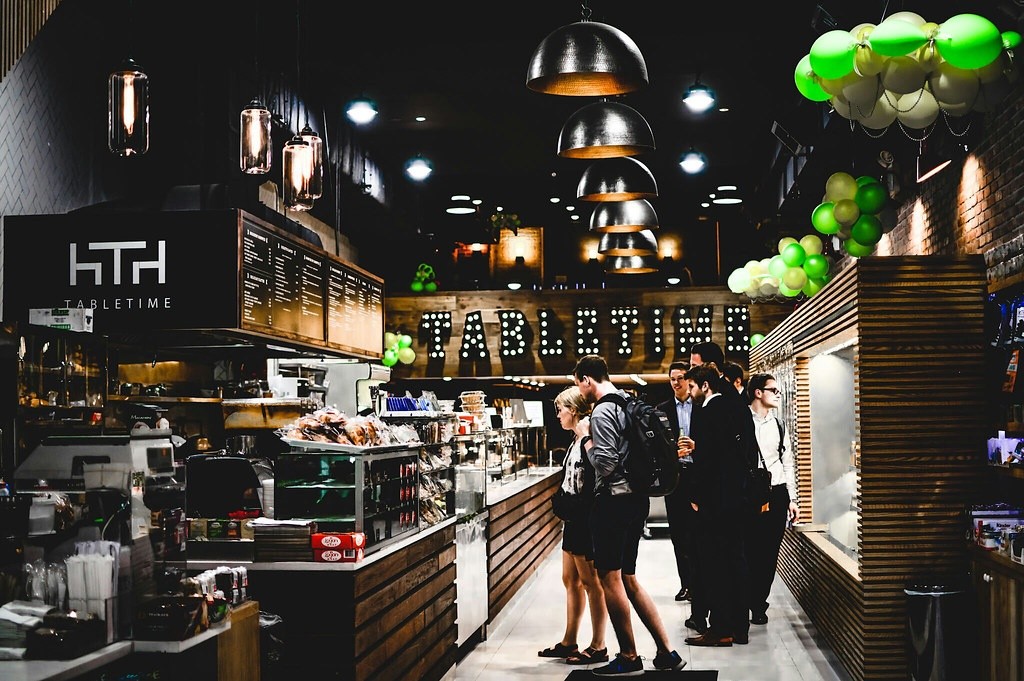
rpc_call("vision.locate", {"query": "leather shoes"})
[675,586,690,600]
[750,605,768,624]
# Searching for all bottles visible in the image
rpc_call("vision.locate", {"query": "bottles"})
[412,511,415,529]
[25,559,67,611]
[400,463,404,484]
[412,486,415,505]
[406,465,410,483]
[412,462,414,481]
[400,486,405,507]
[678,427,687,459]
[405,512,410,531]
[406,485,411,505]
[400,512,404,532]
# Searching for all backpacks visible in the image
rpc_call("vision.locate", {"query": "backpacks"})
[588,389,678,497]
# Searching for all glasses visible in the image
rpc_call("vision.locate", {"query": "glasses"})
[760,388,782,395]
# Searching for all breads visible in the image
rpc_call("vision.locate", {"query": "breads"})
[287,409,386,447]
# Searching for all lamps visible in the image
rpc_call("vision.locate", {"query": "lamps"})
[242,97,322,210]
[347,94,433,183]
[916,150,953,183]
[680,83,714,173]
[526,0,662,272]
[108,58,148,155]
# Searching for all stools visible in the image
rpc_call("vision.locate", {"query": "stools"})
[909,577,972,681]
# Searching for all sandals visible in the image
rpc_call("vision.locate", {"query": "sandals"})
[538,643,579,656]
[566,647,609,664]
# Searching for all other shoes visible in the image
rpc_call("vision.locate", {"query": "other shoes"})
[728,629,748,644]
[685,618,706,632]
[685,628,732,646]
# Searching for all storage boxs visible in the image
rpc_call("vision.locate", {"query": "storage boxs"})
[459,391,488,412]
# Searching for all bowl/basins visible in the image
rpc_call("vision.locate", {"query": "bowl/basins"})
[458,391,487,404]
[459,404,488,415]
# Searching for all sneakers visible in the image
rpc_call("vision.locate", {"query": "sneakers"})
[653,651,687,671]
[592,653,644,676]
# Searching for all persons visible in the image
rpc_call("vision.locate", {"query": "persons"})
[655,341,799,648]
[534,355,688,676]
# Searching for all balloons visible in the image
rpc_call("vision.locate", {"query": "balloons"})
[812,172,898,257]
[750,334,764,346]
[382,332,416,367]
[728,235,836,297]
[794,11,1024,128]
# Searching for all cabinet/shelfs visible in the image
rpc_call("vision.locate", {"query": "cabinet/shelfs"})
[420,444,455,531]
[276,451,420,555]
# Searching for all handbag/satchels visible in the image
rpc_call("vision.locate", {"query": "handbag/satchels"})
[551,489,586,523]
[746,468,773,501]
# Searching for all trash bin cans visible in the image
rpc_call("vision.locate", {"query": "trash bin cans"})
[904,585,978,681]
